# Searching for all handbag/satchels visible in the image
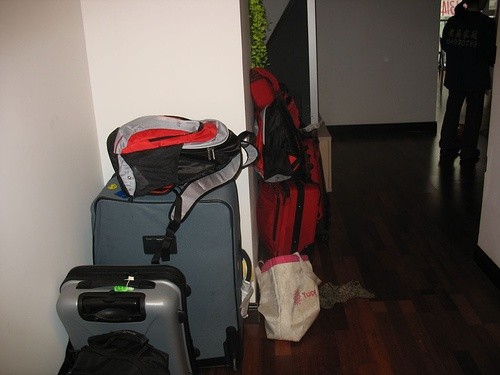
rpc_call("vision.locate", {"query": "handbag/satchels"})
[255,252,322,342]
[251,66,282,108]
[286,96,300,130]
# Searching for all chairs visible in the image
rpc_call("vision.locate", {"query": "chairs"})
[438,37,447,94]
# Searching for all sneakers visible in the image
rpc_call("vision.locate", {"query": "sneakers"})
[460,149,480,160]
[440,145,459,161]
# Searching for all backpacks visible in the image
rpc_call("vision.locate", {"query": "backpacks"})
[106,115,260,265]
[254,100,304,183]
[64,330,171,375]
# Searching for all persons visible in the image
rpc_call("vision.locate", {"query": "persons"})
[439,0,497,168]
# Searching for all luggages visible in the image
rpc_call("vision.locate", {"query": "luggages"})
[258,175,321,257]
[89,171,252,375]
[55,265,200,375]
[301,131,324,224]
